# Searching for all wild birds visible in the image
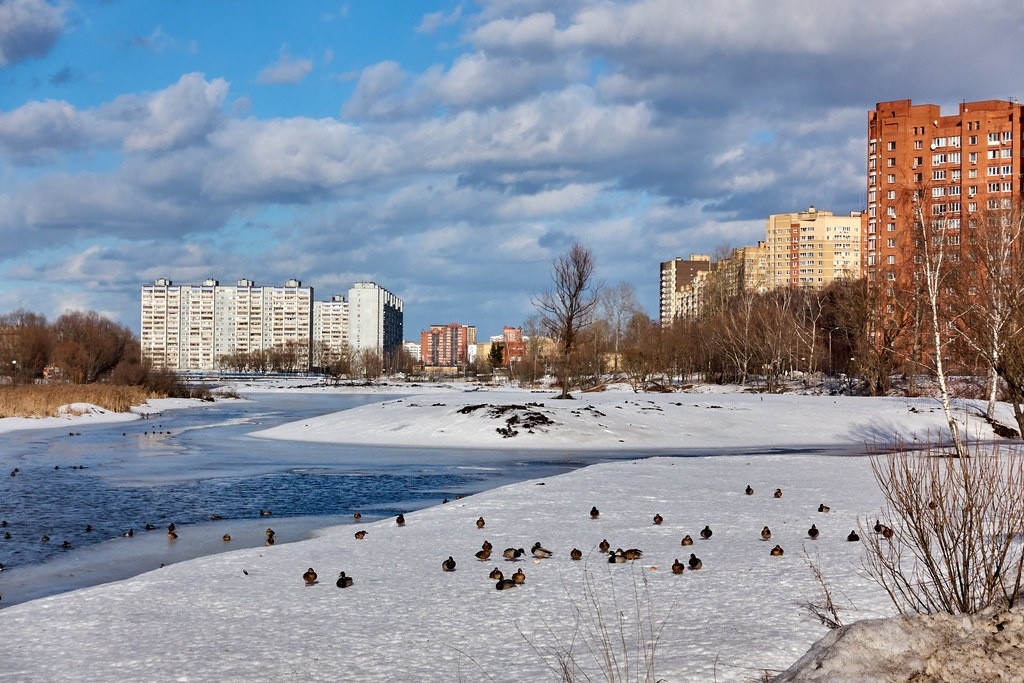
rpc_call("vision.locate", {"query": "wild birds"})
[10,467,19,476]
[166,523,178,541]
[55,465,83,470]
[123,528,134,537]
[63,541,70,548]
[144,431,170,435]
[3,520,7,525]
[209,511,368,545]
[69,432,81,435]
[86,525,91,531]
[145,523,155,529]
[303,567,353,588]
[0,563,5,572]
[123,433,126,435]
[396,514,405,527]
[745,487,939,558]
[443,495,714,591]
[152,425,162,427]
[5,532,9,538]
[42,535,48,541]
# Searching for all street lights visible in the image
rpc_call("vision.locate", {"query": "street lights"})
[821,327,839,368]
